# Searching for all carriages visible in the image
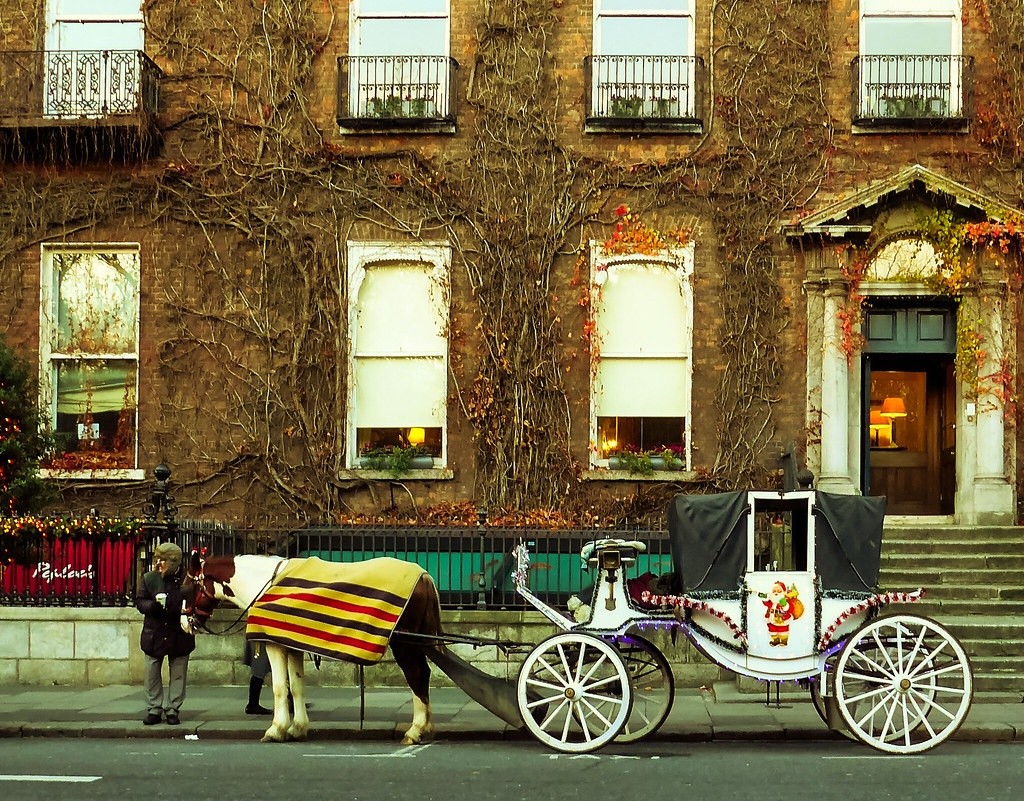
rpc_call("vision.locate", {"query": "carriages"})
[179,467,975,754]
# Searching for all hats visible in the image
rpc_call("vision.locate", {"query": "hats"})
[152,543,183,575]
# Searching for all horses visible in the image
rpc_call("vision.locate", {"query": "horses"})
[180,547,446,746]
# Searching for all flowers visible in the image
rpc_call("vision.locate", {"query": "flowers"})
[404,95,437,118]
[644,97,677,100]
[609,92,645,116]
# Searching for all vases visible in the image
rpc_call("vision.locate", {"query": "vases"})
[645,100,678,118]
[404,101,444,117]
[609,101,642,116]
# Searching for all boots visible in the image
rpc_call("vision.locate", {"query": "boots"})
[286,675,314,714]
[245,676,273,715]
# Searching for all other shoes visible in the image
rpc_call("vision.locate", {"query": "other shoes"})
[166,714,180,725]
[143,714,163,725]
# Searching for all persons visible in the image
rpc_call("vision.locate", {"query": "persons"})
[136,543,196,725]
[242,633,315,715]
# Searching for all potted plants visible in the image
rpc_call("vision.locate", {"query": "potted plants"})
[926,96,948,116]
[360,94,403,119]
[608,447,686,476]
[360,444,434,478]
[882,94,925,117]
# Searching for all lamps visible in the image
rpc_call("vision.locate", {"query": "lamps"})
[409,428,425,443]
[882,396,908,446]
[870,411,892,446]
